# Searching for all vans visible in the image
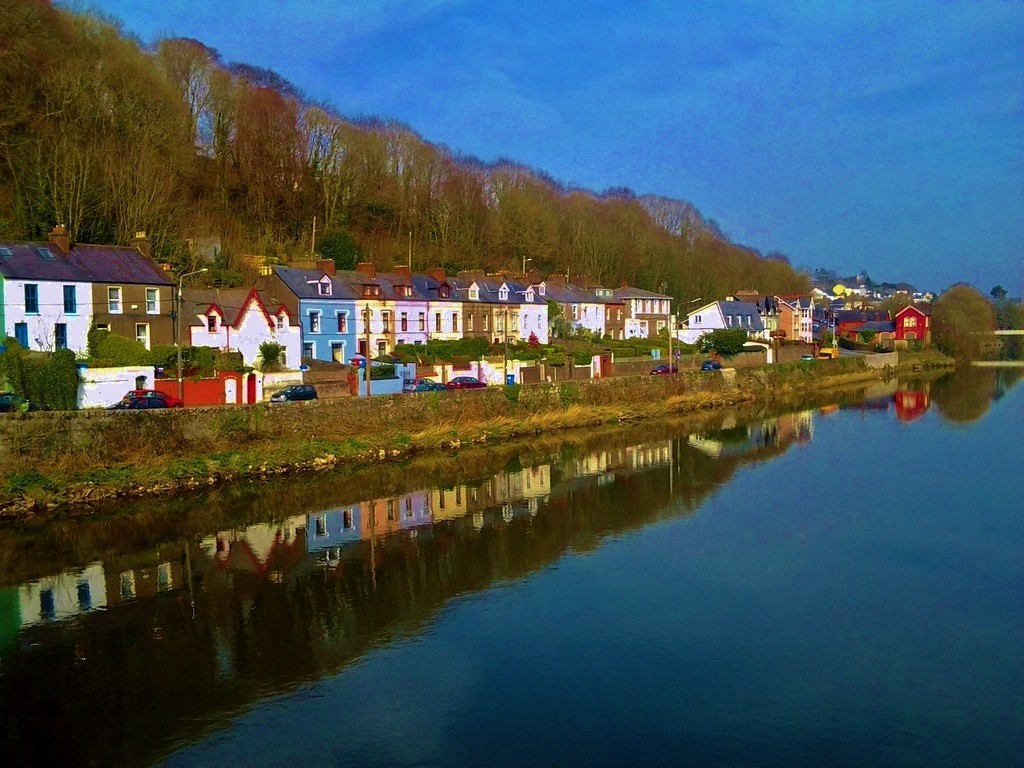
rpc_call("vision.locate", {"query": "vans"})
[701,359,723,371]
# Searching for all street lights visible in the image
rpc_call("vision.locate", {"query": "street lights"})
[177,267,209,400]
[675,297,701,373]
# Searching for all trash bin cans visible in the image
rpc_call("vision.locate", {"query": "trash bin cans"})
[506,374,515,385]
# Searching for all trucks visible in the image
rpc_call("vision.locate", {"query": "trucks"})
[817,348,839,360]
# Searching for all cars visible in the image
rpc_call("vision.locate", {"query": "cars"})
[269,384,319,403]
[650,364,678,375]
[0,389,52,412]
[122,390,184,409]
[445,377,488,389]
[402,377,446,392]
[103,396,168,410]
[800,354,814,361]
